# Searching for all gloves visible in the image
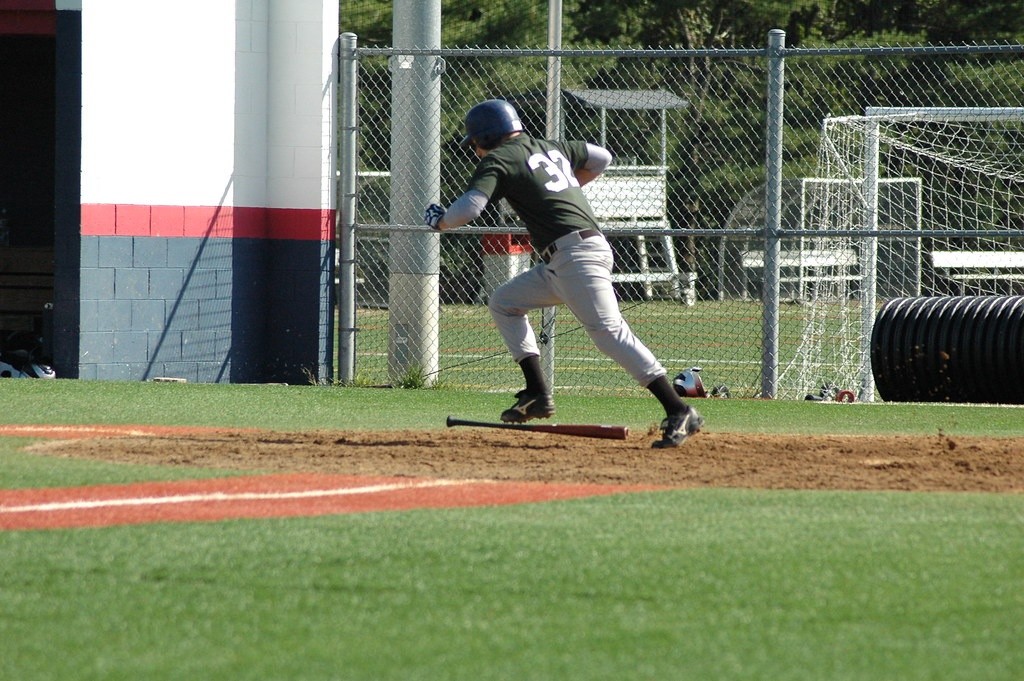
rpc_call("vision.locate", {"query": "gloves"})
[424,204,448,231]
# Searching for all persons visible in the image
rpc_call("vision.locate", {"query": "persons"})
[424,97,707,451]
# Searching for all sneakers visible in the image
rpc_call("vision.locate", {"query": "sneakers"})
[500,387,555,423]
[652,405,705,448]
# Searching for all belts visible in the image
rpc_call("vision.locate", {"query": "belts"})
[543,230,601,264]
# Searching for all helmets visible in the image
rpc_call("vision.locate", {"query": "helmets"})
[460,99,523,150]
[673,369,708,397]
